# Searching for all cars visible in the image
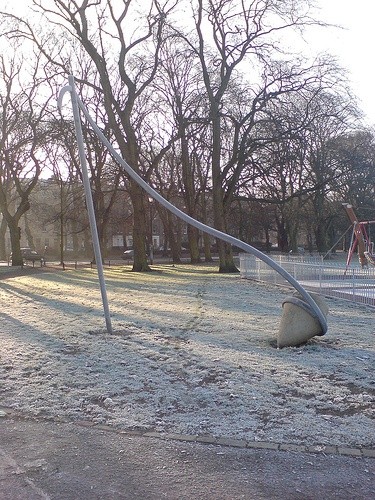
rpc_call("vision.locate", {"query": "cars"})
[9,248,43,261]
[122,250,134,259]
